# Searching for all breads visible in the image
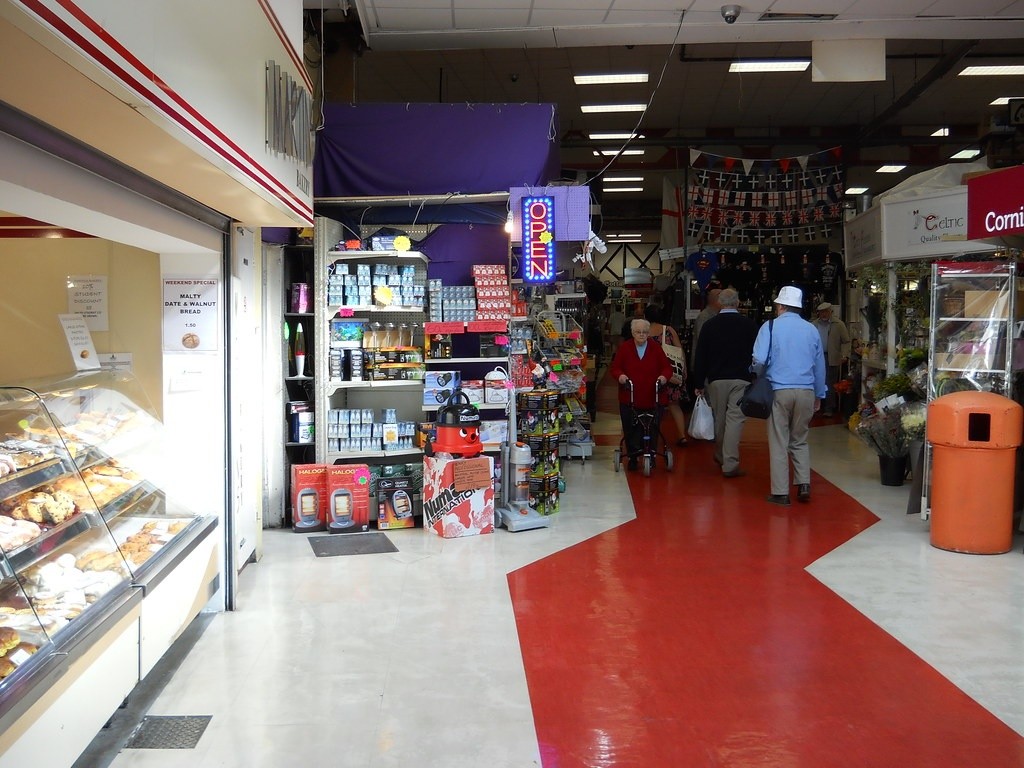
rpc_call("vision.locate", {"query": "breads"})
[1,424,187,683]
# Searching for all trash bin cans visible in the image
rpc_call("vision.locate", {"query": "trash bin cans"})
[928,391,1024,557]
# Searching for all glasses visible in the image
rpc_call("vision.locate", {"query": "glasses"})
[635,331,649,334]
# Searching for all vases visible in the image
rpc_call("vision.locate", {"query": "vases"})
[873,453,908,486]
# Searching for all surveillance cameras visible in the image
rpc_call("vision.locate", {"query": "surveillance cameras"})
[721,5,741,24]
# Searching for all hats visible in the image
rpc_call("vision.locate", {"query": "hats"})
[774,285,803,308]
[816,302,831,310]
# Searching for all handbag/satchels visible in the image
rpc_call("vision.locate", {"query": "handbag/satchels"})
[736,376,775,419]
[688,394,715,440]
[661,323,683,386]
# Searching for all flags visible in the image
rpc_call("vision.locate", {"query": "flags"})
[688,164,844,244]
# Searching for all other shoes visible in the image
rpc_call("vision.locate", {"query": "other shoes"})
[822,411,833,417]
[723,472,745,478]
[627,457,639,470]
[798,483,810,500]
[768,494,790,503]
[677,437,688,446]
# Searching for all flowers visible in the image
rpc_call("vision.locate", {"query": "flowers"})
[853,399,926,454]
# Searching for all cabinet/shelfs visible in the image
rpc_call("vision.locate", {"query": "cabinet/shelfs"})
[512,310,537,393]
[321,220,427,475]
[918,257,1024,523]
[546,290,602,463]
[423,318,514,501]
[529,312,592,442]
[282,220,318,451]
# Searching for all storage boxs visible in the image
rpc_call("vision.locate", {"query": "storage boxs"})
[964,288,1024,319]
[949,339,1024,369]
[933,351,1004,369]
[283,235,565,538]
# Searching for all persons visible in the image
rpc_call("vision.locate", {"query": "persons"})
[811,302,850,418]
[749,286,828,507]
[610,303,688,471]
[690,289,760,477]
